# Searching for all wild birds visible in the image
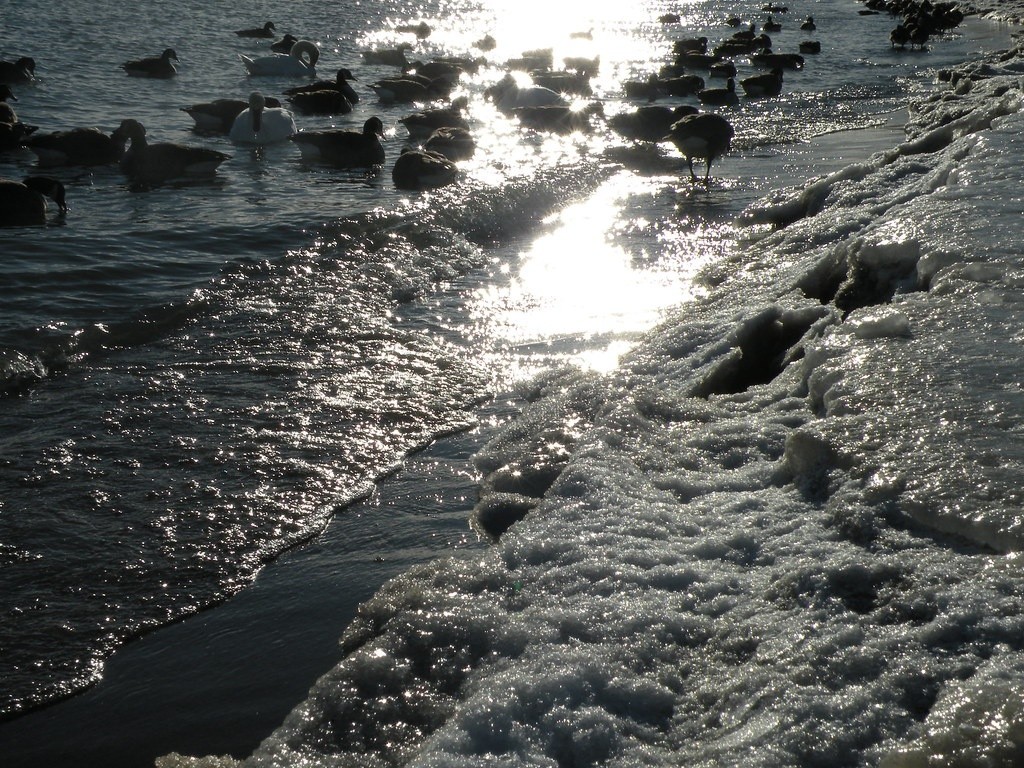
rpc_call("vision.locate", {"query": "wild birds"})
[858,0,966,52]
[1,0,822,227]
